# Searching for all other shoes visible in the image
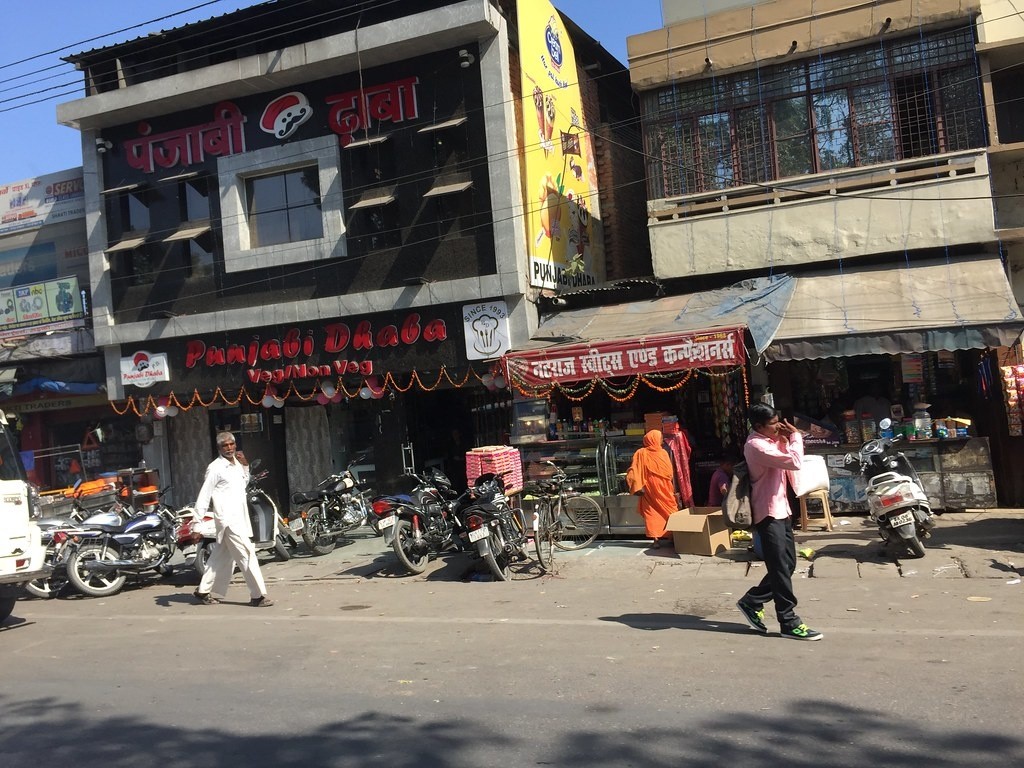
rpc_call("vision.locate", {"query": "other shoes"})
[251,596,274,607]
[192,586,223,604]
[652,544,661,549]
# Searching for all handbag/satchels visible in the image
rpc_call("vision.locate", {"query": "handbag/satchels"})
[786,454,830,497]
[722,460,753,531]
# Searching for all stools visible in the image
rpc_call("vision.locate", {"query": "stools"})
[800,489,834,532]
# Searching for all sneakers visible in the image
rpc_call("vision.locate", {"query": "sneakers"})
[735,598,768,635]
[779,623,824,640]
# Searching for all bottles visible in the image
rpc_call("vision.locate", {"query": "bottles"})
[548,404,609,440]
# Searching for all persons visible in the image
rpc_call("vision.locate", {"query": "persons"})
[708,453,733,506]
[736,402,824,641]
[192,431,274,607]
[626,430,679,549]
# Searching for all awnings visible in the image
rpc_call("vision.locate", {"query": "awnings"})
[506,258,1024,389]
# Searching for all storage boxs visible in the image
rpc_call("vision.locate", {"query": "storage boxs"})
[664,506,731,556]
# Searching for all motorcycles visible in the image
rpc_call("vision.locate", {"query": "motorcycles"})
[457,471,528,582]
[19,479,178,599]
[842,418,937,558]
[174,458,290,580]
[291,454,386,555]
[368,466,457,574]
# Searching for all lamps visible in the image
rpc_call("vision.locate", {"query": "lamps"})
[96,138,112,153]
[6,404,16,418]
[459,50,475,68]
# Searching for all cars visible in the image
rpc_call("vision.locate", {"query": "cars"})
[0,408,55,622]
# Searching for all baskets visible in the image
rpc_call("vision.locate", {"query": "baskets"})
[562,479,582,497]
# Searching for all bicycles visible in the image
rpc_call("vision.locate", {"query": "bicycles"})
[530,458,604,575]
